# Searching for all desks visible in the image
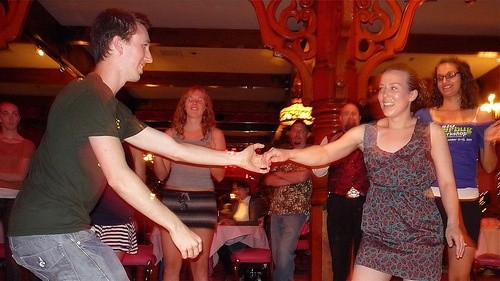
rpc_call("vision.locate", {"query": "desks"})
[474,218,500,259]
[151,226,270,281]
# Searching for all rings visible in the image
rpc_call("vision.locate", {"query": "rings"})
[463,242,467,245]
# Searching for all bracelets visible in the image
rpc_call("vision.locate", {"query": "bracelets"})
[224,150,236,165]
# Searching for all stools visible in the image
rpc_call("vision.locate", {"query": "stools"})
[231,248,274,281]
[474,253,500,281]
[123,245,160,281]
[296,222,310,250]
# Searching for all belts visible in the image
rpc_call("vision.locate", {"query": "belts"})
[347,187,367,199]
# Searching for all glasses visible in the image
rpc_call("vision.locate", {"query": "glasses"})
[436,71,461,81]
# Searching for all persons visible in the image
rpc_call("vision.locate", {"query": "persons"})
[312,102,373,281]
[263,61,467,281]
[151,85,224,281]
[9,8,273,281]
[218,179,261,281]
[413,56,500,281]
[264,120,314,281]
[90,132,146,263]
[0,99,37,281]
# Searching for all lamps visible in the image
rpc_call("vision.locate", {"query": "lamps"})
[279,96,314,127]
